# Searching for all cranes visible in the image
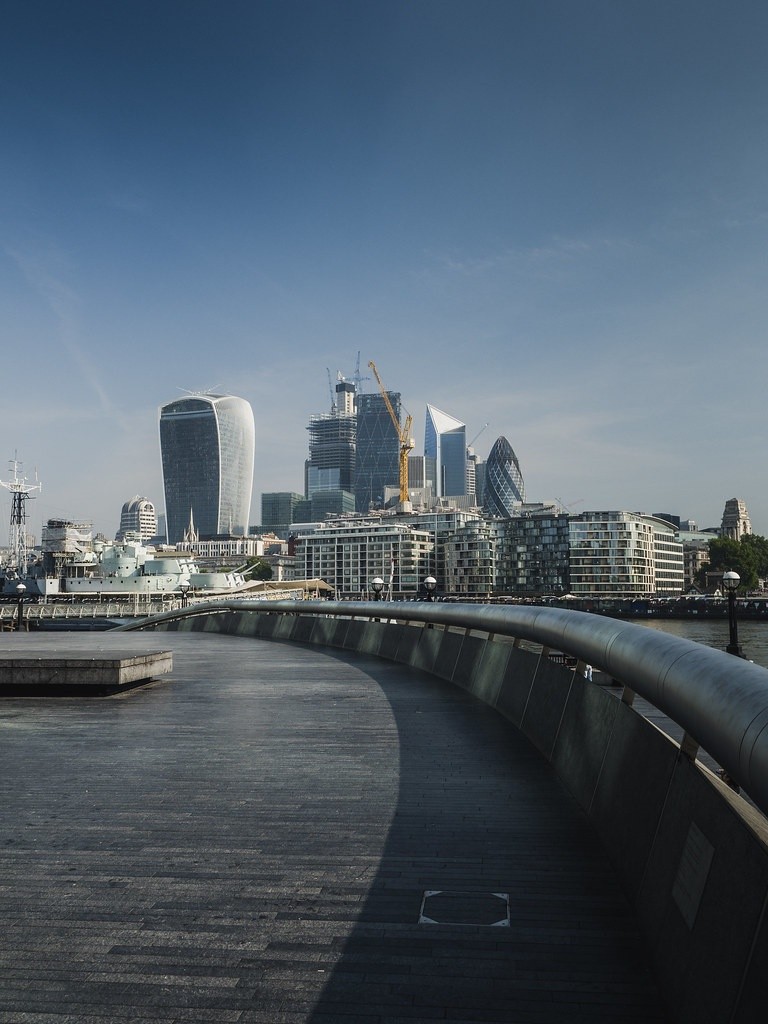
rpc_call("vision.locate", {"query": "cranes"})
[367,359,416,514]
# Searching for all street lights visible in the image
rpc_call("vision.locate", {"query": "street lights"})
[371,578,384,622]
[16,583,26,632]
[721,572,747,659]
[179,580,191,608]
[424,576,438,628]
